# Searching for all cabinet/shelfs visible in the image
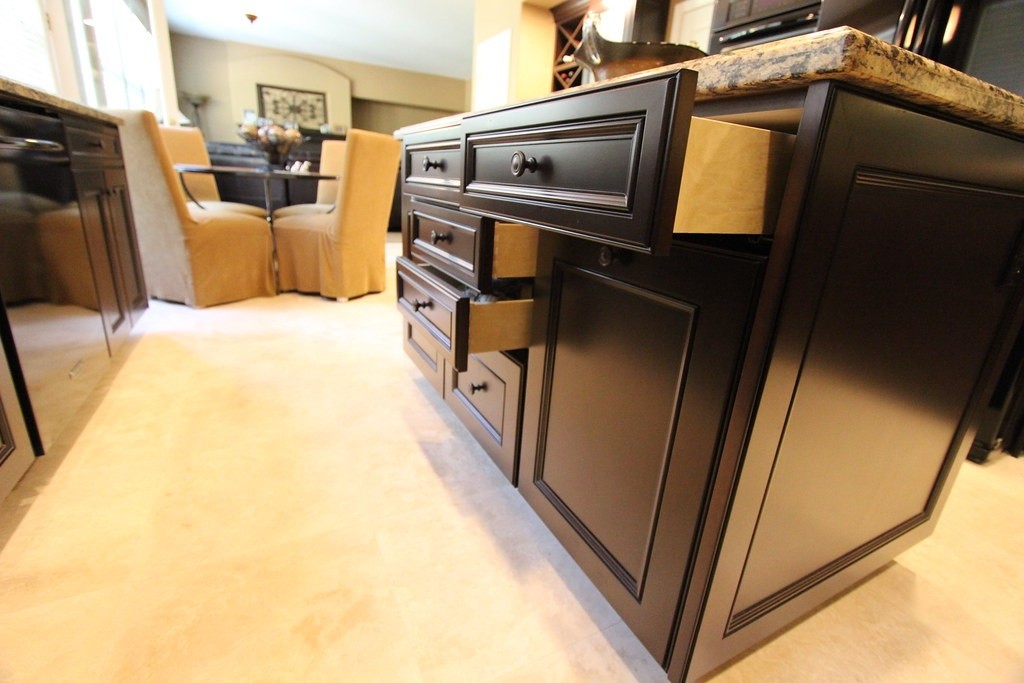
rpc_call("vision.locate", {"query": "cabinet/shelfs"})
[0,90,148,508]
[394,65,1024,683]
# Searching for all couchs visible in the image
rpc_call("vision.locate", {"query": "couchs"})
[203,143,320,206]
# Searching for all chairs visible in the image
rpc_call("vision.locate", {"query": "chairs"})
[105,109,274,308]
[270,129,402,304]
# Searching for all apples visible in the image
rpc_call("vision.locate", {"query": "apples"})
[241,122,301,147]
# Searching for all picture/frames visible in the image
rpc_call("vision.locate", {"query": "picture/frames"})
[257,83,328,128]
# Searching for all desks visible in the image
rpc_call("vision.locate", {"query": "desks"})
[178,165,337,223]
